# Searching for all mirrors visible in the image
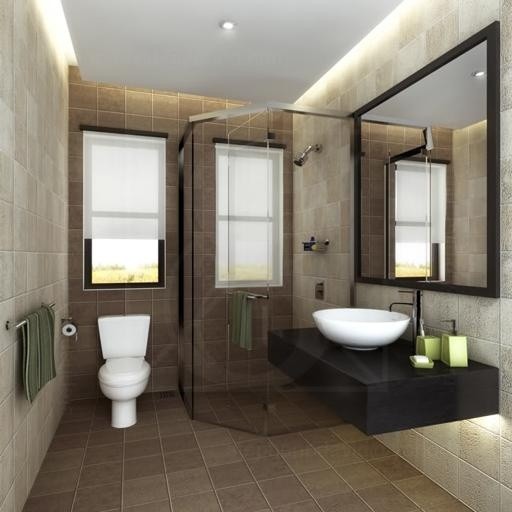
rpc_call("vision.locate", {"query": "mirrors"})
[354,21,500,298]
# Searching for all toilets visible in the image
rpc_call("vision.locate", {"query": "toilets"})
[97,315,153,429]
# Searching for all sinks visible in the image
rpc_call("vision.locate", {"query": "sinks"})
[312,306,412,352]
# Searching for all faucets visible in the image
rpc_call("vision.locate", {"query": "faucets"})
[389,288,423,349]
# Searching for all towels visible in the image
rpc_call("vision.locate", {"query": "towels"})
[230,291,252,351]
[21,304,56,404]
